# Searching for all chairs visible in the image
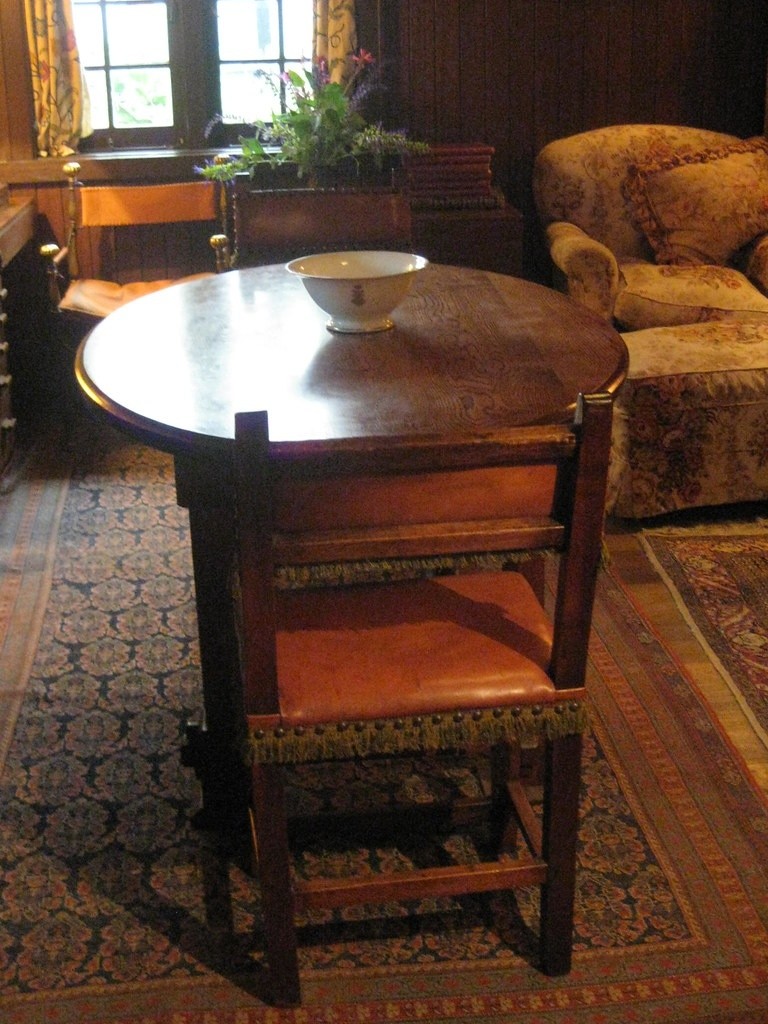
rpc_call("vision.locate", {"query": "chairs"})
[39,151,232,475]
[232,388,614,1007]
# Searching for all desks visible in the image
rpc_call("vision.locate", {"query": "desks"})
[73,263,631,930]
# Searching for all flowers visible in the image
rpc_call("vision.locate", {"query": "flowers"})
[190,45,432,184]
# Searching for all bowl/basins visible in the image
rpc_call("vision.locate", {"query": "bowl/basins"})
[284,250,429,333]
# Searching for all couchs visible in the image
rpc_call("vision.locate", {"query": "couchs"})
[532,123,768,526]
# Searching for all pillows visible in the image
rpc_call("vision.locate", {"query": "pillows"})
[613,256,768,331]
[625,133,767,267]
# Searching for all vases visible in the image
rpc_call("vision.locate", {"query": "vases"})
[232,153,416,272]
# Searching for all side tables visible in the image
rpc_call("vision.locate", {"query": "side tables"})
[409,201,526,283]
